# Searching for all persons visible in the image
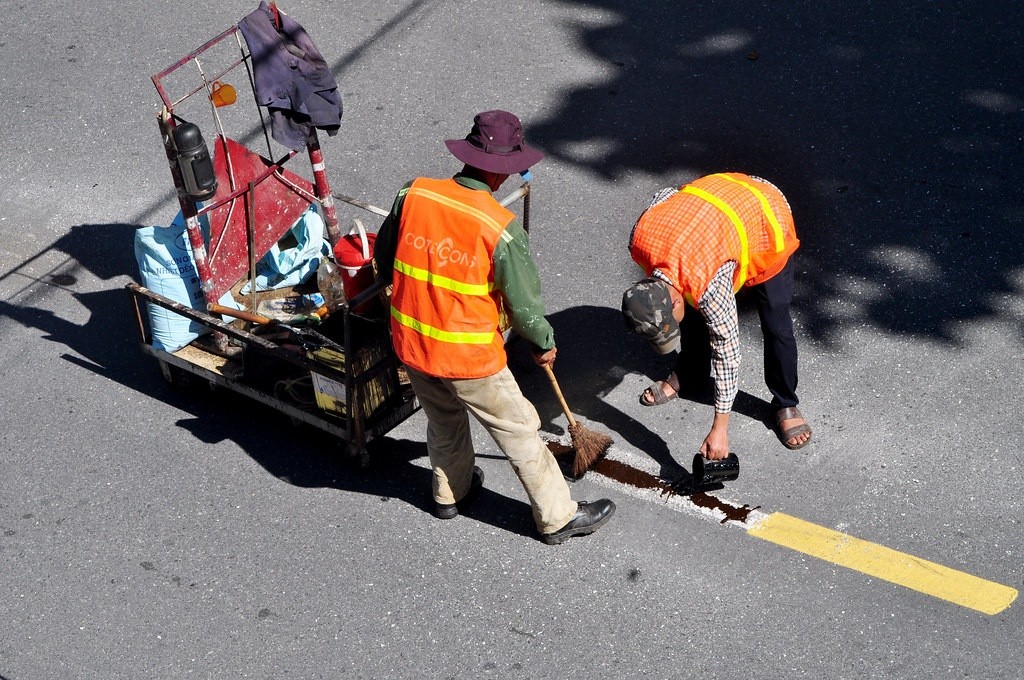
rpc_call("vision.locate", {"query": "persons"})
[372,110,617,546]
[621,171,814,460]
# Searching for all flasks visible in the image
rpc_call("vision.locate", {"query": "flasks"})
[172,123,218,202]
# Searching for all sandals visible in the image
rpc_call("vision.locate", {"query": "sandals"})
[639,372,680,406]
[774,406,812,450]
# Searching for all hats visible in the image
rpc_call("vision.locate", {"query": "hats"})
[445,110,546,175]
[621,278,682,355]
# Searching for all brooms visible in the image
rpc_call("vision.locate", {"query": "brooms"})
[542,363,616,481]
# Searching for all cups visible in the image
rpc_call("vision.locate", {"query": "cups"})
[693,452,739,485]
[208,81,237,108]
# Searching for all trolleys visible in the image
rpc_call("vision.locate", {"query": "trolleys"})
[126,2,531,466]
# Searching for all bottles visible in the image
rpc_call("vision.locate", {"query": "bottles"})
[317,256,347,313]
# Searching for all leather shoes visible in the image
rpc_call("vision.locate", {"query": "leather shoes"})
[433,465,484,519]
[546,498,617,545]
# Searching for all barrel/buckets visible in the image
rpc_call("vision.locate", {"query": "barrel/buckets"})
[333,219,380,313]
